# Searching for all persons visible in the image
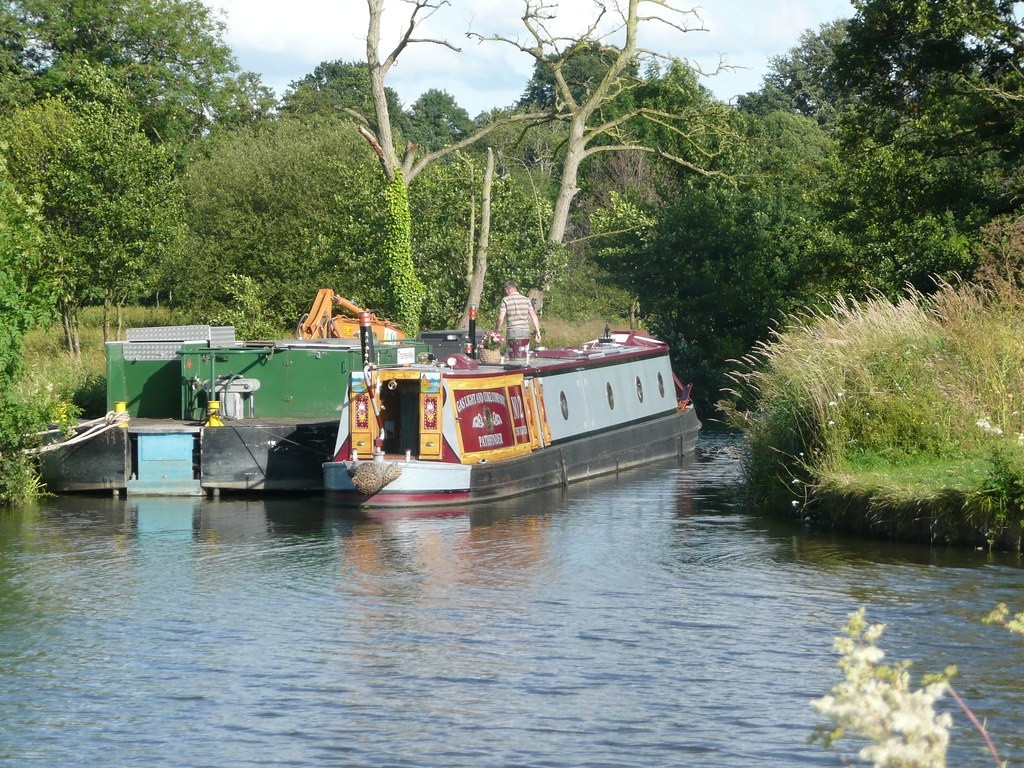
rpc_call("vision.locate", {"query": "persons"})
[495,281,542,360]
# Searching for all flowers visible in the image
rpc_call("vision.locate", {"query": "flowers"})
[477,329,506,351]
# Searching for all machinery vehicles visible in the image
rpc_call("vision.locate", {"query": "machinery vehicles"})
[292,286,411,346]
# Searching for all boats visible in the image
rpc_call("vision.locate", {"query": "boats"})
[321,302,710,511]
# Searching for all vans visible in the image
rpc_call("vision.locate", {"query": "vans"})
[413,329,490,362]
